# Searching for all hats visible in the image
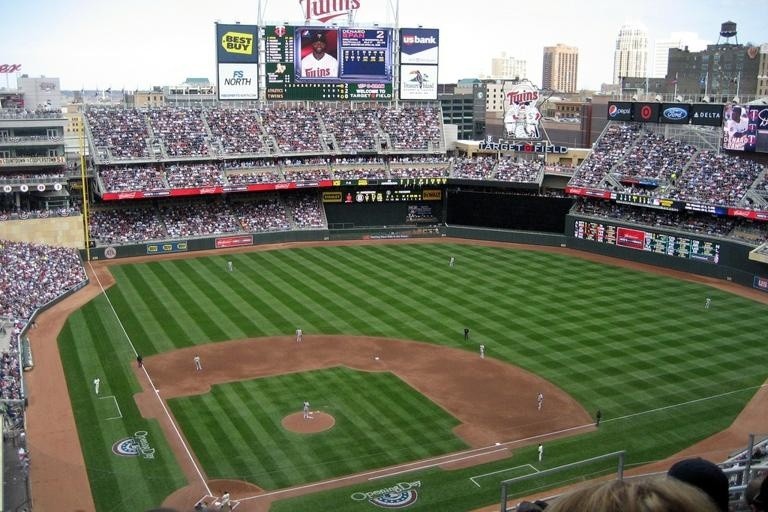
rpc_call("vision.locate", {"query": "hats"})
[667,458,729,506]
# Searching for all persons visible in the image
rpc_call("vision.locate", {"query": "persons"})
[220,492,232,510]
[301,33,338,77]
[295,327,303,343]
[194,502,203,512]
[135,354,143,367]
[464,327,469,342]
[518,442,768,512]
[479,344,486,359]
[705,296,712,308]
[0,105,767,247]
[227,258,233,270]
[450,256,455,265]
[193,354,203,369]
[1,241,88,434]
[92,377,102,396]
[596,408,603,426]
[303,400,309,419]
